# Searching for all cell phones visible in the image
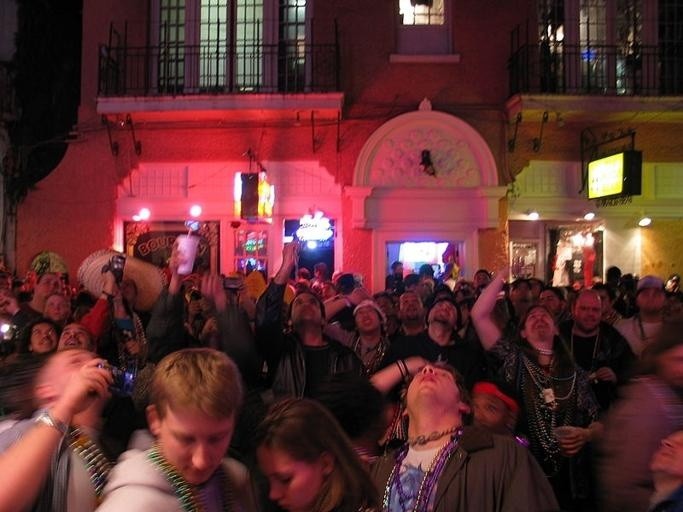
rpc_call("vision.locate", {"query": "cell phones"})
[191,290,202,300]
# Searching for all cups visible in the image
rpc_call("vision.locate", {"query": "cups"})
[176,233,201,277]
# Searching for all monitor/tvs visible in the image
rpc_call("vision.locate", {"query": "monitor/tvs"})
[233,171,275,219]
[587,151,642,199]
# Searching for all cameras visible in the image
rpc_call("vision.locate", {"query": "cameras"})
[99,363,136,397]
[102,255,126,283]
[513,264,535,279]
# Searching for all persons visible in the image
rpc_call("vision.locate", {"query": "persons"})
[0,242,683,512]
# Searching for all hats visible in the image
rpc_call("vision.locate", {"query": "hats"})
[636,274,665,293]
